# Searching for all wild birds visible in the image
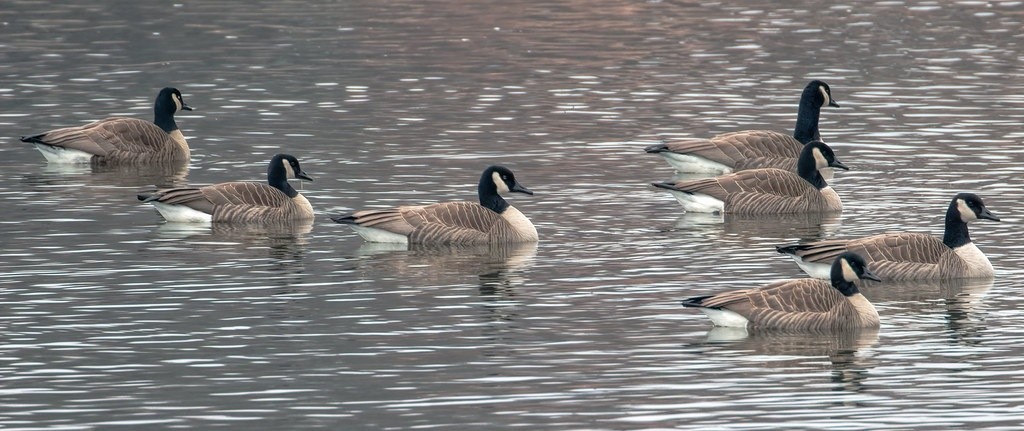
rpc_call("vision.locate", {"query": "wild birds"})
[330,165,539,242]
[680,252,882,330]
[643,79,840,173]
[775,194,1001,280]
[137,154,314,222]
[650,141,850,211]
[18,87,192,162]
